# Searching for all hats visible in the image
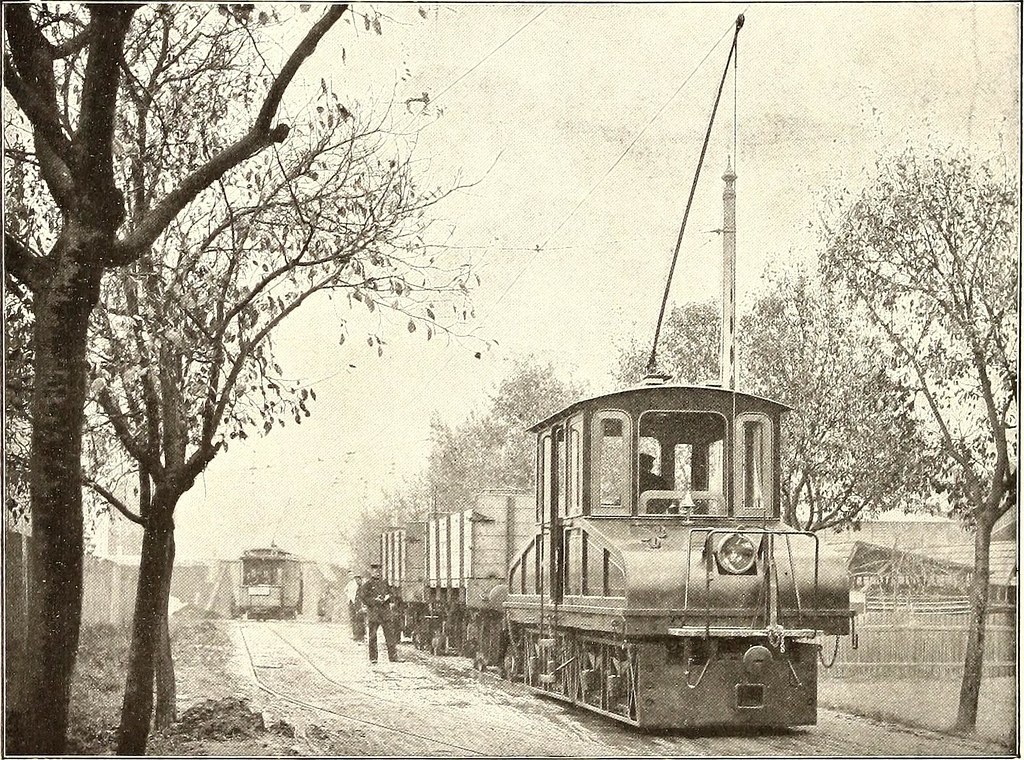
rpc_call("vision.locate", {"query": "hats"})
[371,562,383,569]
[640,454,654,464]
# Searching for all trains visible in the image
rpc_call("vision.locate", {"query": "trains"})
[380,384,860,733]
[238,547,304,622]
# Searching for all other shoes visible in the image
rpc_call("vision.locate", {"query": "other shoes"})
[371,659,377,663]
[390,657,406,662]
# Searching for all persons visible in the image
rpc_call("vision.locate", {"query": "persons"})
[616,454,672,516]
[347,572,367,643]
[322,584,335,622]
[360,562,406,663]
